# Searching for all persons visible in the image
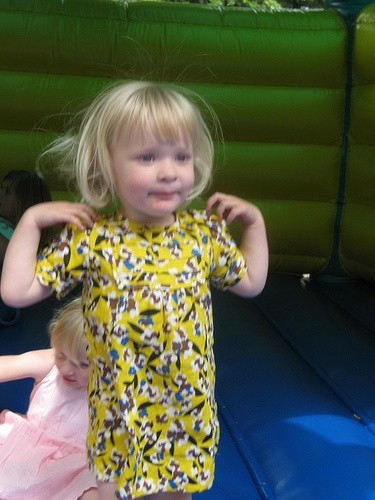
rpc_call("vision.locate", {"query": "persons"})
[0,78,269,500]
[0,168,52,290]
[0,296,102,500]
